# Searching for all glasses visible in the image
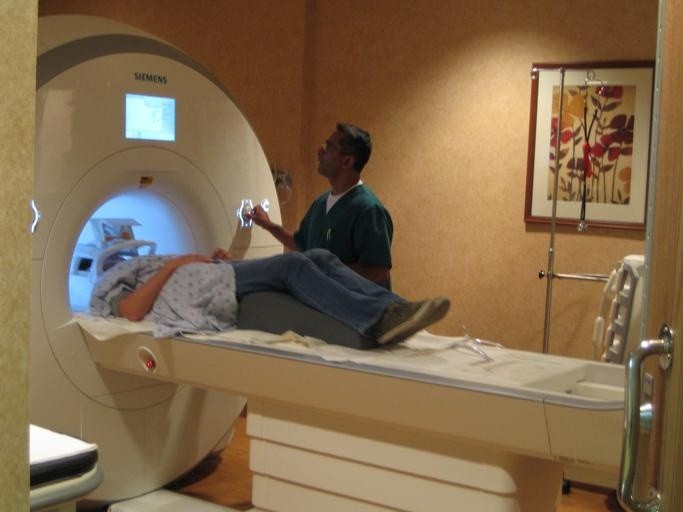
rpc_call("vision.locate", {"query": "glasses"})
[322,140,342,155]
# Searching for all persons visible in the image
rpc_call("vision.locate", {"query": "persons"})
[245,119,395,293]
[88,240,449,350]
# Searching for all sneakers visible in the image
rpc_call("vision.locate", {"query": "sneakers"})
[372,298,435,344]
[425,297,451,323]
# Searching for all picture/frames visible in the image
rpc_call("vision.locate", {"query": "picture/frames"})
[523,58,655,233]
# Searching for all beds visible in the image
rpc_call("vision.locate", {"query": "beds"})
[75,307,635,474]
